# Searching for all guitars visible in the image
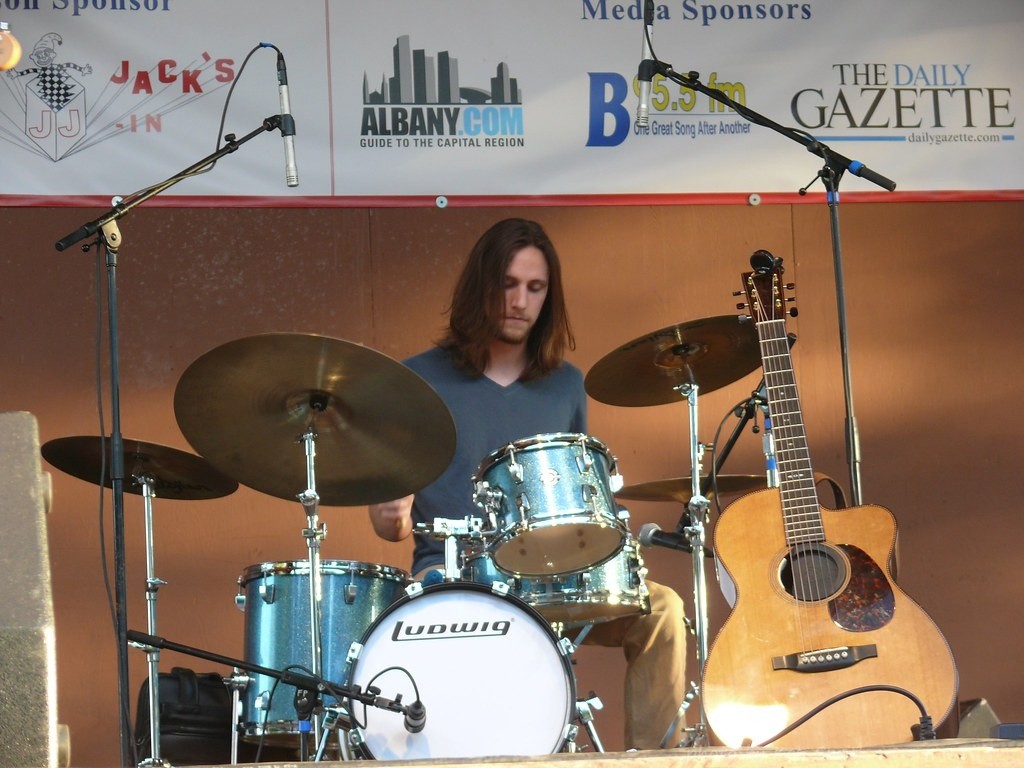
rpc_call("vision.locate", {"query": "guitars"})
[701,248,961,750]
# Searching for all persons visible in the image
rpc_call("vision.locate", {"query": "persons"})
[365,217,688,750]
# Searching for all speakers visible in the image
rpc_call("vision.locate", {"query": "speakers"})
[934,698,1000,739]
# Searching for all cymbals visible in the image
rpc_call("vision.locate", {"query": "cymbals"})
[42,435,240,502]
[172,331,455,504]
[607,474,769,503]
[584,314,762,408]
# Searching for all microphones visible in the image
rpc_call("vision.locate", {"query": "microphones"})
[636,0,656,127]
[762,413,780,489]
[637,522,713,558]
[403,700,427,733]
[277,51,299,187]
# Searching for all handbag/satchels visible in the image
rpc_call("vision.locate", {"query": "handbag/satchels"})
[135,666,300,763]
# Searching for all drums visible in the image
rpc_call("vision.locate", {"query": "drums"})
[237,558,414,751]
[341,579,576,762]
[465,532,654,634]
[473,431,630,580]
